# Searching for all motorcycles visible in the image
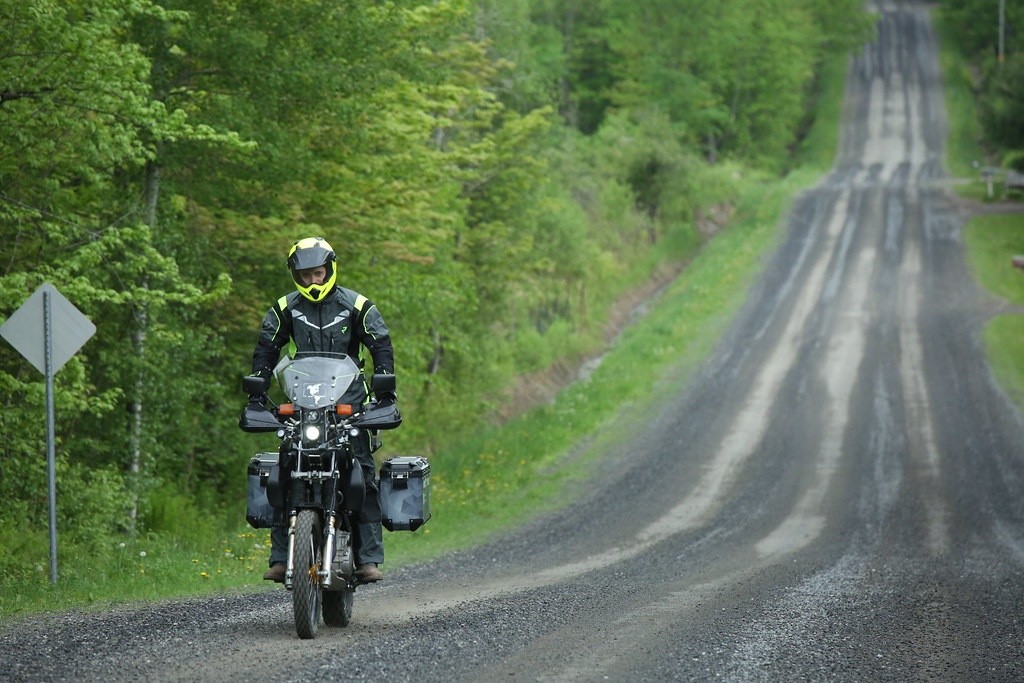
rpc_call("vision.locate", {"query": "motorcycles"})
[240,351,432,637]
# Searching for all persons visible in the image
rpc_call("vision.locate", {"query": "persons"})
[250,237,396,582]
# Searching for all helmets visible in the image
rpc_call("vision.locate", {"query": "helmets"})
[285,237,338,304]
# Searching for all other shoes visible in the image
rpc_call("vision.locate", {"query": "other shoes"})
[264,562,286,580]
[355,562,383,582]
